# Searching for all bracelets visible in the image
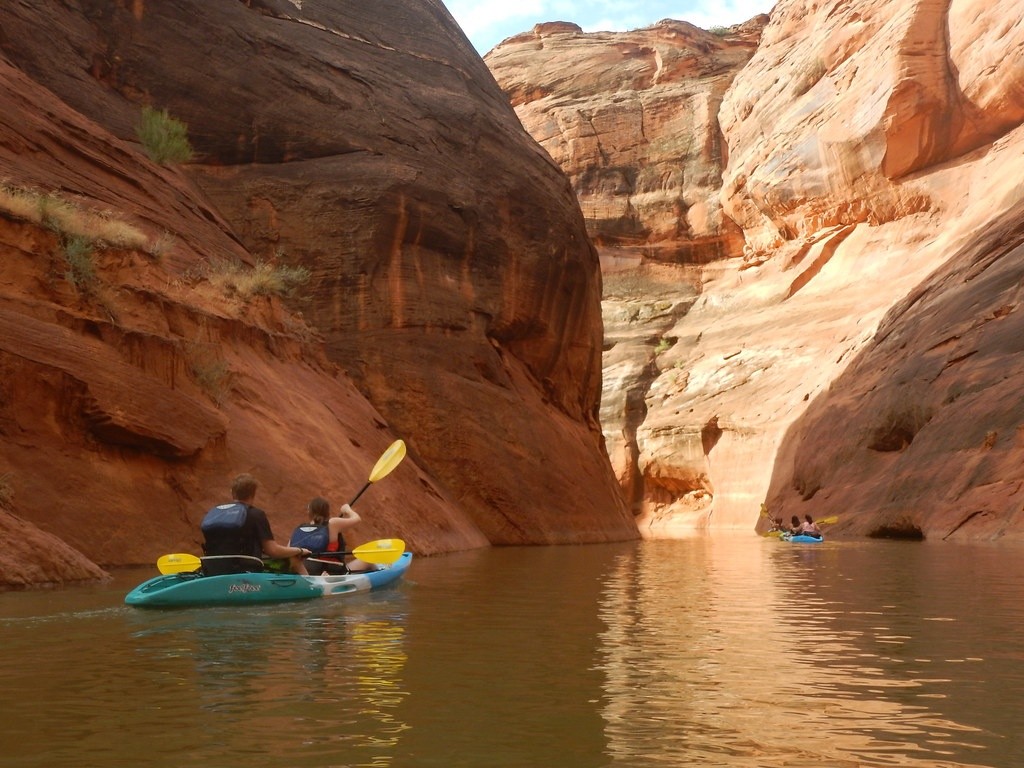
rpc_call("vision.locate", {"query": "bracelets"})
[296,547,303,556]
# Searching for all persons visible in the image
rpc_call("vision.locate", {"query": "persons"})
[790,516,803,534]
[768,518,786,532]
[790,514,824,537]
[286,495,378,575]
[201,473,312,576]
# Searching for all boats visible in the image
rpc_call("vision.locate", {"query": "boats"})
[762,531,784,537]
[779,532,824,543]
[124,550,413,606]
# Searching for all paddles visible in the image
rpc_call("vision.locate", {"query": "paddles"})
[760,502,781,523]
[156,536,406,575]
[766,516,838,535]
[337,438,406,517]
